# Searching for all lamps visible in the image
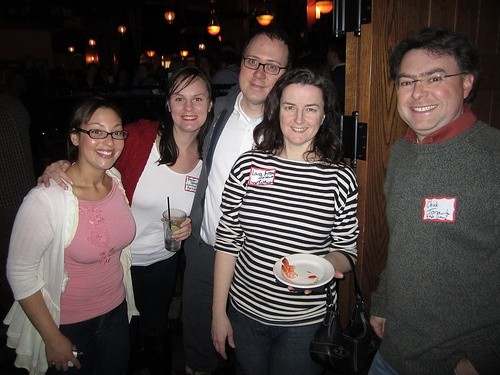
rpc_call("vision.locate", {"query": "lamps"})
[145,0,274,58]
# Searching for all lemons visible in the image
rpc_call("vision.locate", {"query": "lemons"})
[171,225,180,230]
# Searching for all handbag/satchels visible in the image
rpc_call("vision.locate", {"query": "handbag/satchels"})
[308,249,381,375]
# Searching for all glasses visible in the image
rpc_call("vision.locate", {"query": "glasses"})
[397,71,470,90]
[241,54,287,75]
[76,127,129,141]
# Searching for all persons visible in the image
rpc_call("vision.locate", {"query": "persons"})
[366,28,500,375]
[36,65,214,375]
[180,26,294,375]
[211,69,358,375]
[3,95,141,375]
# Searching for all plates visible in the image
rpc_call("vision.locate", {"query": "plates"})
[273,253,335,289]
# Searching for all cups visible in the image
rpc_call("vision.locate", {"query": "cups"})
[162,208,187,252]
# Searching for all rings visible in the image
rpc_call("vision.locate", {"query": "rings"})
[187,230,191,234]
[42,173,50,176]
